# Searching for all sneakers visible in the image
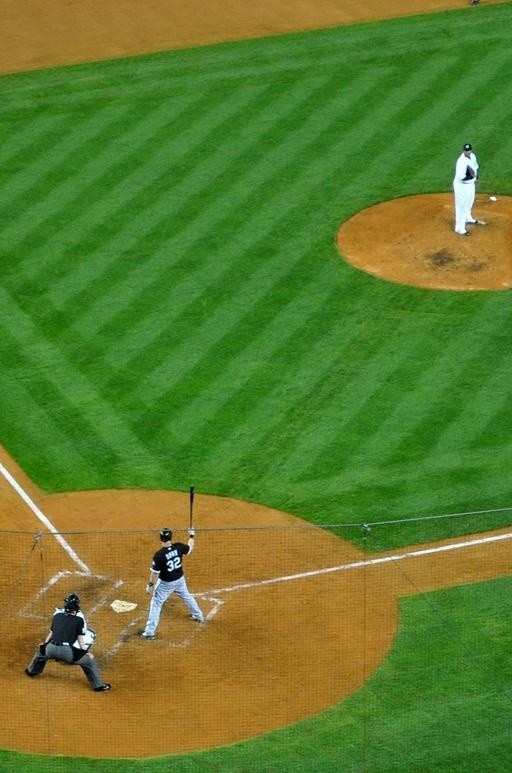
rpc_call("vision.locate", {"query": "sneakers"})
[94,684,110,691]
[138,631,155,640]
[468,219,478,224]
[189,614,205,623]
[457,229,470,236]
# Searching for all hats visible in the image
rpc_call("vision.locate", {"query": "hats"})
[464,144,473,152]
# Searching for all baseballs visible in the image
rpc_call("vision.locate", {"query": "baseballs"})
[491,197,496,200]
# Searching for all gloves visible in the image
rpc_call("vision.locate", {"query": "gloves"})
[146,582,153,595]
[187,527,195,538]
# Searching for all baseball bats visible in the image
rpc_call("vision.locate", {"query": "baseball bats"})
[189,485,193,529]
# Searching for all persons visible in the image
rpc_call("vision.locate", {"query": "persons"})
[136,525,205,639]
[450,141,480,237]
[24,600,112,693]
[51,591,97,653]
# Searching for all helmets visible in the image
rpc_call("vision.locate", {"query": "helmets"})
[159,528,171,543]
[64,593,80,615]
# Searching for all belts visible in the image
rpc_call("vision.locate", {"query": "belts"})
[51,640,68,646]
